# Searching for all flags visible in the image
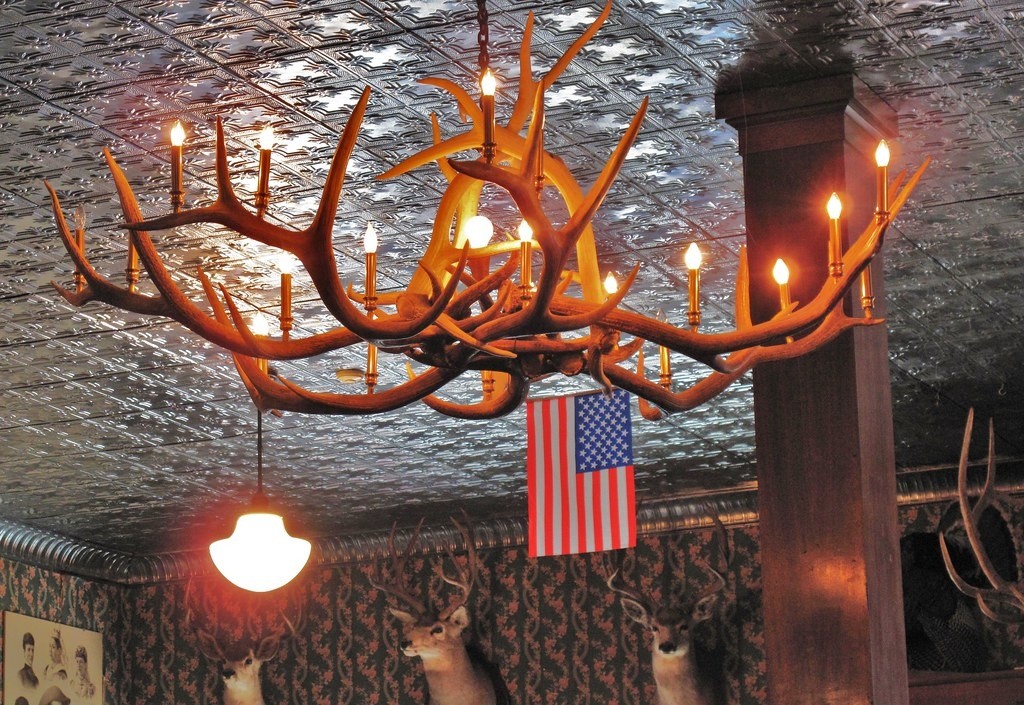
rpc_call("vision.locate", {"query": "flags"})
[527,389,637,557]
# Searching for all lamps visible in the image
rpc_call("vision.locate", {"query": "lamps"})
[209,366,311,594]
[41,0,931,422]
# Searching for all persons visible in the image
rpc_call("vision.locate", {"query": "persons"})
[15,632,95,705]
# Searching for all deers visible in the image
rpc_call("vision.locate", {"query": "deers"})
[367,508,502,705]
[183,571,306,705]
[606,495,731,705]
